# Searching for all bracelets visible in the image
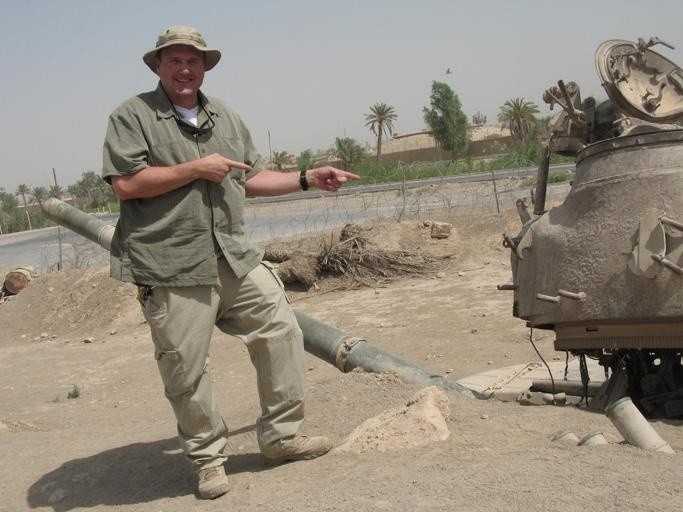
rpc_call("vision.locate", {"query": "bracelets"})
[298,168,308,193]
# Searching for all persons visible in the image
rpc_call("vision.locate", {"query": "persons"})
[100,24,363,500]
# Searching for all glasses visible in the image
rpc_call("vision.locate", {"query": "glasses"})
[170,102,216,136]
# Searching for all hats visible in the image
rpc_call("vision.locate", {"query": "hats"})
[141,26,222,75]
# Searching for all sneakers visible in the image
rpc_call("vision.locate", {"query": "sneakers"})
[197,465,231,500]
[262,434,333,467]
